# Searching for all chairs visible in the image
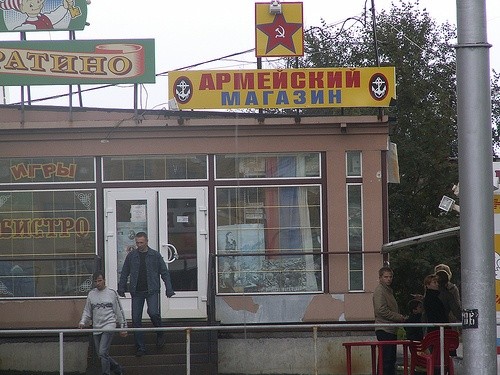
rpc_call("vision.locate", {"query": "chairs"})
[409,329,460,375]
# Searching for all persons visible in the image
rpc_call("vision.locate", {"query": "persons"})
[372,268,410,375]
[403,300,426,362]
[78,273,129,375]
[118,231,175,356]
[423,262,463,375]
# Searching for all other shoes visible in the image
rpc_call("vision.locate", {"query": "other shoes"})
[103,373,109,375]
[117,367,125,375]
[157,334,162,344]
[136,350,144,356]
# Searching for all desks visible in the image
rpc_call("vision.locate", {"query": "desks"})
[342,340,411,375]
